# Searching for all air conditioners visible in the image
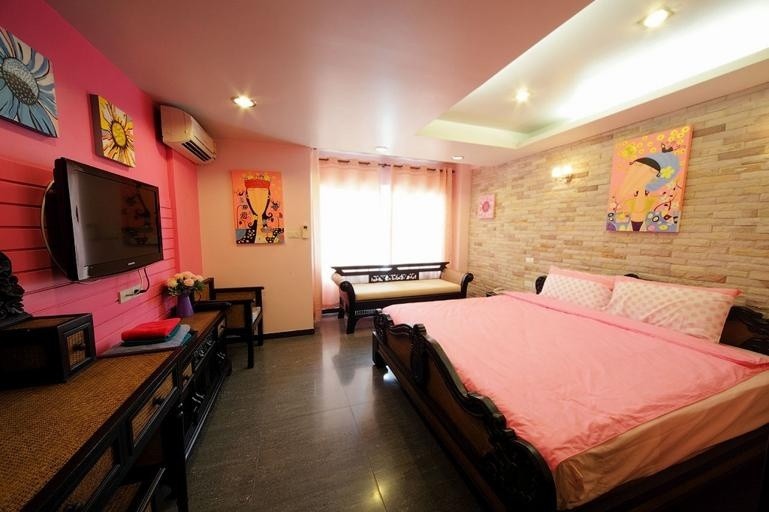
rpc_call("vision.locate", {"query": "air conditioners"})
[159,101,215,166]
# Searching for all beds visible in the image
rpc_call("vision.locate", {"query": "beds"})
[373,265,767,506]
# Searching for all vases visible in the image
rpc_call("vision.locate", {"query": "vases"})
[174,294,193,316]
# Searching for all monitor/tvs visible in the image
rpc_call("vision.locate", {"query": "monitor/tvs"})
[45,157,164,282]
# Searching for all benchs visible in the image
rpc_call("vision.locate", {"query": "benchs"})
[330,262,472,335]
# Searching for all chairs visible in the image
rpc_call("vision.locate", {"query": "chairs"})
[196,278,266,369]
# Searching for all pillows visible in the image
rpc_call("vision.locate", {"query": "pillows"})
[536,265,614,314]
[604,273,742,343]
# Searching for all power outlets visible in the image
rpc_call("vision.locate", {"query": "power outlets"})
[118,283,144,304]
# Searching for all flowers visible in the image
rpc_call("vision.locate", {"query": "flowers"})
[165,270,205,297]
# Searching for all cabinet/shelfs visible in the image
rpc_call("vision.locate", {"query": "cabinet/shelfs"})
[1,310,235,512]
